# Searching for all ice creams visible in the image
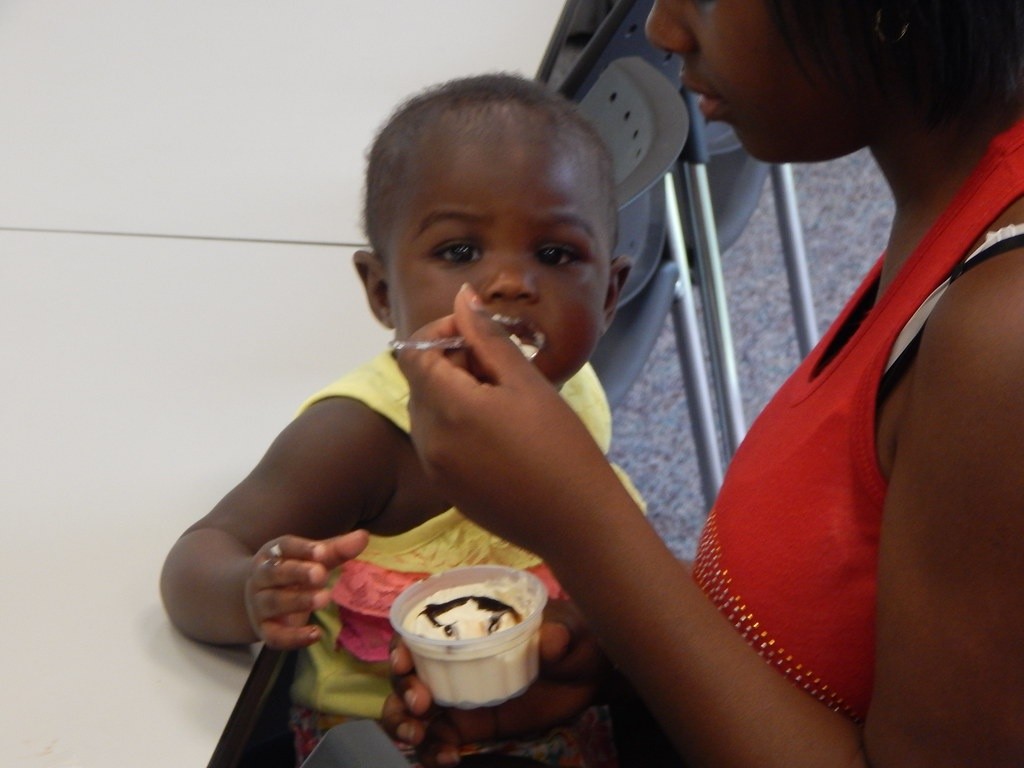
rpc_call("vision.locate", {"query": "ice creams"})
[389,565,548,709]
[509,333,539,361]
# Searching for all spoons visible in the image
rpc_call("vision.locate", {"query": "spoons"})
[389,333,541,363]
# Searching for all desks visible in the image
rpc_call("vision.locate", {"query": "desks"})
[0,1,566,768]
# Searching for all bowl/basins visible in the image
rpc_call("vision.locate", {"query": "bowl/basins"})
[388,565,548,711]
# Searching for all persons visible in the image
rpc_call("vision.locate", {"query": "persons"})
[396,0,1024,768]
[158,72,649,768]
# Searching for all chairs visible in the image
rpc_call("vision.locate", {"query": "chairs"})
[544,0,818,512]
